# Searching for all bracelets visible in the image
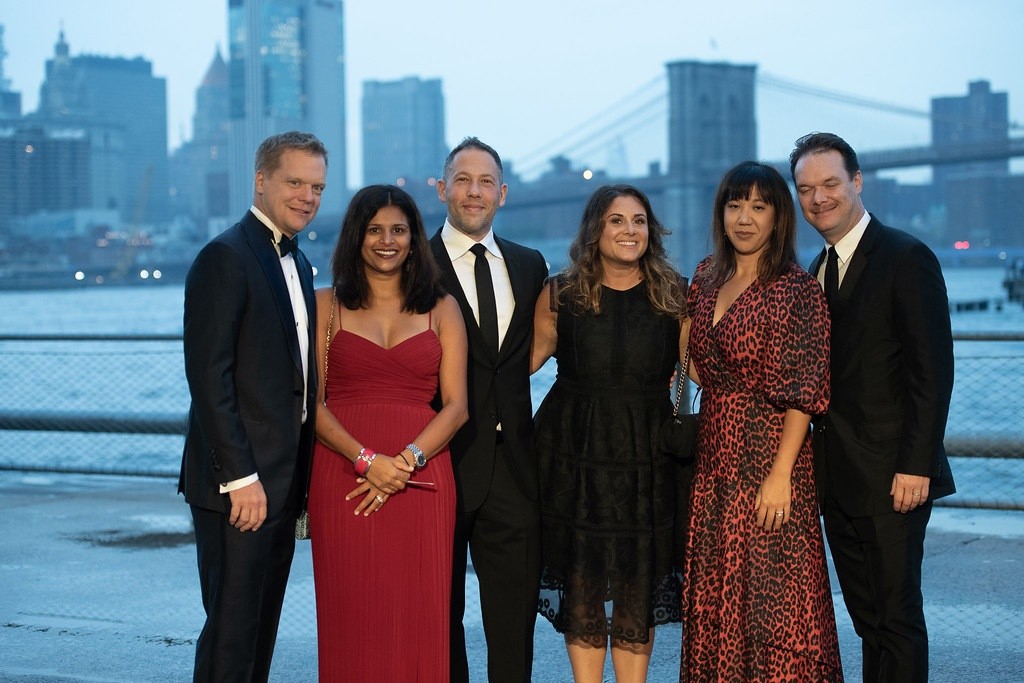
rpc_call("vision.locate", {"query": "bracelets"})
[396,452,411,468]
[353,447,377,476]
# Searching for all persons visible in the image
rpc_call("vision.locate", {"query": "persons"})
[789,132,957,683]
[429,137,677,683]
[177,130,328,683]
[530,185,702,683]
[679,162,846,683]
[308,186,470,683]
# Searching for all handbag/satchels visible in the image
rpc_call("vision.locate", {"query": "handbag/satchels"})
[294,509,310,540]
[659,414,700,458]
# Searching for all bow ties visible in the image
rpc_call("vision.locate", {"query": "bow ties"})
[277,233,298,259]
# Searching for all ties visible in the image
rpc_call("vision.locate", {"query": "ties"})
[824,246,839,306]
[468,243,499,365]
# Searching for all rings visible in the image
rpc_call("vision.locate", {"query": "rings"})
[912,492,921,497]
[774,510,784,517]
[375,495,384,504]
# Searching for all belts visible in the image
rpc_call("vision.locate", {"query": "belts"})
[495,431,504,446]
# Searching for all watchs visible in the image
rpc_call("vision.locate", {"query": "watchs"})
[406,443,427,471]
